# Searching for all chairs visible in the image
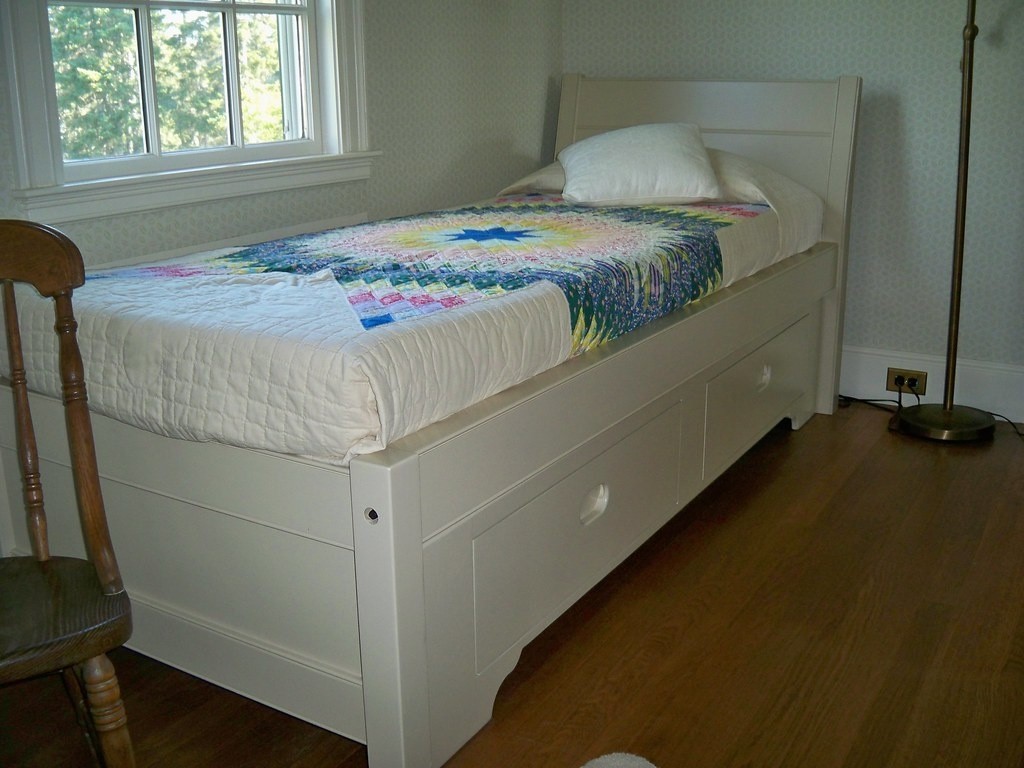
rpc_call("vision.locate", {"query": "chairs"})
[0,218,139,767]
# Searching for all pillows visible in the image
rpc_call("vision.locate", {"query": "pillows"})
[495,147,789,202]
[557,121,722,207]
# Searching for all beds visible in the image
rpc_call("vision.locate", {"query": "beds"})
[0,70,864,768]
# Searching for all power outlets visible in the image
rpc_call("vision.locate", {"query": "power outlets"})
[886,367,928,395]
